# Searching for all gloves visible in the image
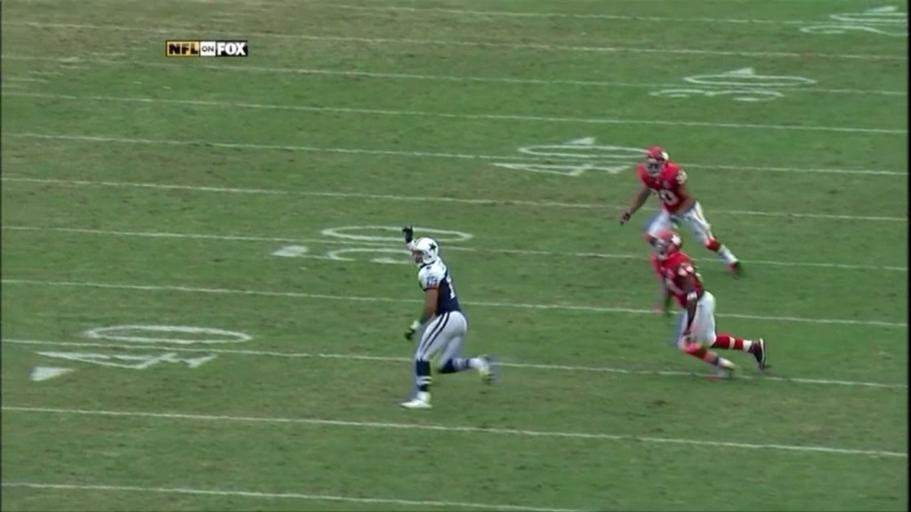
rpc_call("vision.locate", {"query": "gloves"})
[403,329,415,341]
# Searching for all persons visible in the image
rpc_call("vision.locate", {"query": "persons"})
[617,144,745,280]
[401,222,498,409]
[643,225,767,381]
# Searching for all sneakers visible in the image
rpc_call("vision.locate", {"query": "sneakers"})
[478,354,494,385]
[399,396,433,409]
[748,338,766,370]
[718,364,737,379]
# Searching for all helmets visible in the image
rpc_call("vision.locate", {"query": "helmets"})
[412,237,440,265]
[645,146,670,167]
[655,228,683,255]
